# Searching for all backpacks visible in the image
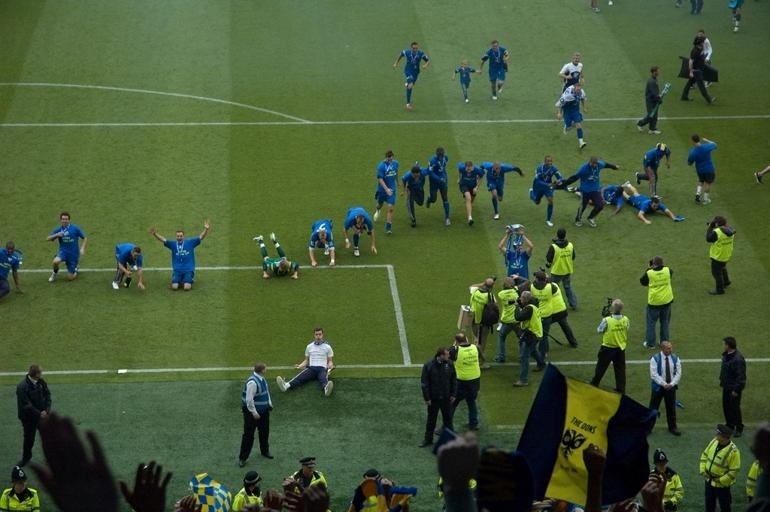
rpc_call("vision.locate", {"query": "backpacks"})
[482,291,499,326]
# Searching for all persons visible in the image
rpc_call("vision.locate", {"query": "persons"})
[753,164,766,186]
[12,361,55,468]
[1,411,173,512]
[0,204,380,297]
[700,215,739,297]
[274,322,338,399]
[437,432,684,512]
[688,134,718,203]
[542,226,579,312]
[530,143,678,227]
[592,254,682,435]
[496,222,534,282]
[381,38,515,110]
[238,362,276,466]
[0,210,210,296]
[552,1,746,149]
[420,269,578,454]
[180,457,417,512]
[700,336,769,512]
[373,147,525,232]
[259,208,377,278]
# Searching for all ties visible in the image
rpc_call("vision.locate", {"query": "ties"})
[665,357,671,384]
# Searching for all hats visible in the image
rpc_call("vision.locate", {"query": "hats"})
[243,471,262,485]
[299,457,316,468]
[363,468,380,481]
[714,424,733,437]
[653,450,669,464]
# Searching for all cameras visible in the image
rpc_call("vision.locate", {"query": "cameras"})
[649,259,652,267]
[706,222,715,228]
[490,275,497,282]
[508,297,521,306]
[533,266,545,276]
[602,297,613,318]
[511,272,520,285]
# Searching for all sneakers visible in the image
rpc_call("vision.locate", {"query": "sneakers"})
[276,376,287,393]
[252,235,264,241]
[694,193,701,202]
[479,353,549,387]
[579,142,587,150]
[637,121,642,133]
[353,248,360,258]
[373,197,499,234]
[270,231,276,241]
[648,130,661,135]
[529,172,662,227]
[112,281,120,290]
[708,288,725,295]
[325,381,334,396]
[702,199,711,205]
[643,341,655,349]
